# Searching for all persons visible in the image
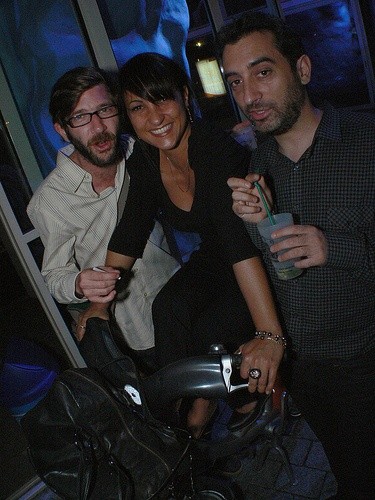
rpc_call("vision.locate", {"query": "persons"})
[27,64,135,305]
[216,12,374,500]
[74,51,287,395]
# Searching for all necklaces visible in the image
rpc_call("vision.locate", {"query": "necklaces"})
[165,155,191,193]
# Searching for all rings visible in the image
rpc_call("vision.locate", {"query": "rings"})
[247,368,261,379]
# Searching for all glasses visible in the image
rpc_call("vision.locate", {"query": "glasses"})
[63,103,121,128]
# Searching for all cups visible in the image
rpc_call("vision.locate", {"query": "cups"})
[257,212,303,282]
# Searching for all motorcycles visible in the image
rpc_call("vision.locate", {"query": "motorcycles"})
[65,318,303,500]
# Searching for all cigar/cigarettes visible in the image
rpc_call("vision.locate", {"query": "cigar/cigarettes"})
[92,267,121,280]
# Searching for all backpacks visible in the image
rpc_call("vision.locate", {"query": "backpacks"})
[19,368,195,500]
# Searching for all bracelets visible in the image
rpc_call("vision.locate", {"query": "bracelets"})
[253,332,287,350]
